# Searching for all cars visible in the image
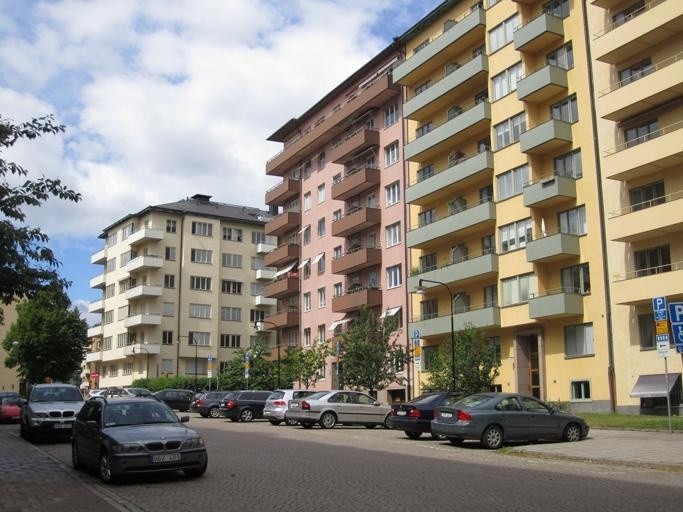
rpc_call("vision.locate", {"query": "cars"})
[72,396,208,482]
[391,393,468,440]
[0,384,85,441]
[286,390,395,429]
[431,391,589,449]
[262,390,317,425]
[123,387,194,412]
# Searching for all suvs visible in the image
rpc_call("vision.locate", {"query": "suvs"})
[189,391,231,418]
[219,390,273,422]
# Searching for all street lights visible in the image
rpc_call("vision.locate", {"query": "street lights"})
[177,335,198,394]
[132,347,149,379]
[417,277,457,390]
[252,320,281,388]
